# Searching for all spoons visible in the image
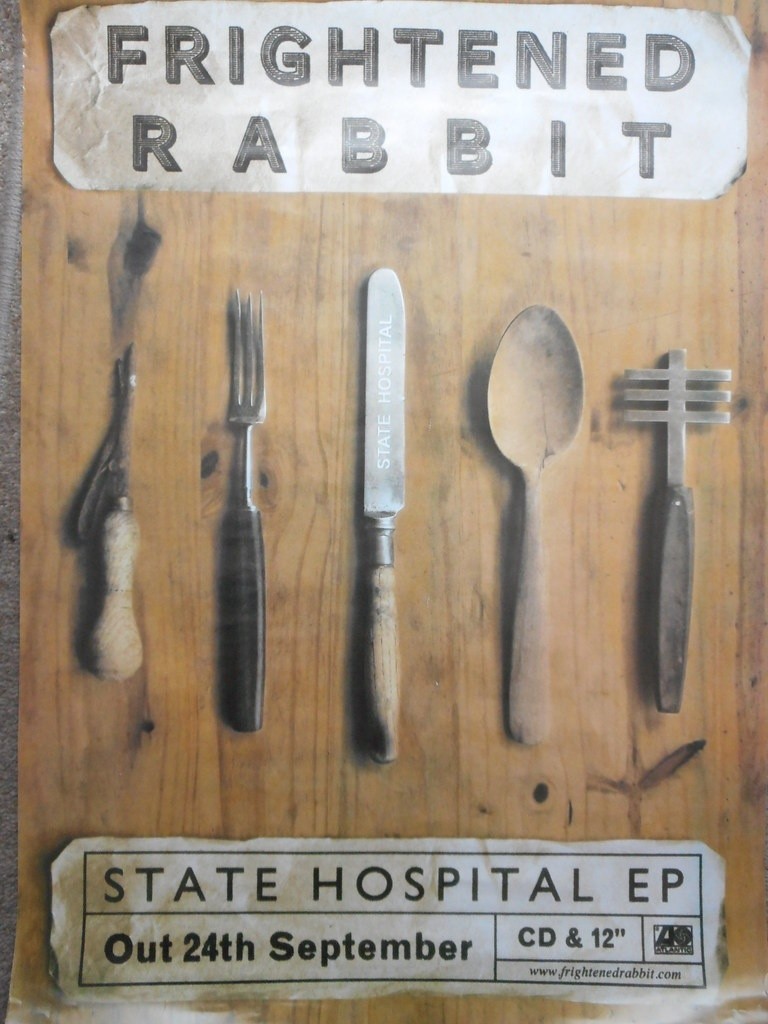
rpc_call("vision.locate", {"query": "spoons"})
[486,305,586,744]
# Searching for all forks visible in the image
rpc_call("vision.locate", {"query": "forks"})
[223,287,269,732]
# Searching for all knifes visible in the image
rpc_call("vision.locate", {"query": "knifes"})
[363,266,407,765]
